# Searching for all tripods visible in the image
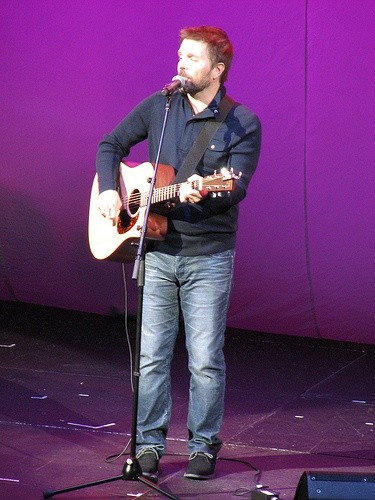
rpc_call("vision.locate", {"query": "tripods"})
[42,94,180,500]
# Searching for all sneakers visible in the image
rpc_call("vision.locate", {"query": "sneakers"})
[136,444,166,476]
[184,451,217,478]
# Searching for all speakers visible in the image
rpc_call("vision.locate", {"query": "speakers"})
[295,470,375,500]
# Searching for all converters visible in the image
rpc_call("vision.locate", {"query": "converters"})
[252,488,280,500]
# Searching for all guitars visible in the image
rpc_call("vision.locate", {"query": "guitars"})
[88,159,243,264]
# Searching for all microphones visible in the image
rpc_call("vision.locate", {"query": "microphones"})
[160,75,184,95]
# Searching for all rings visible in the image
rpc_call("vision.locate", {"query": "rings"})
[191,201,195,203]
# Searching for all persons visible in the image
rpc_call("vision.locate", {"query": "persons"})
[95,25,261,481]
